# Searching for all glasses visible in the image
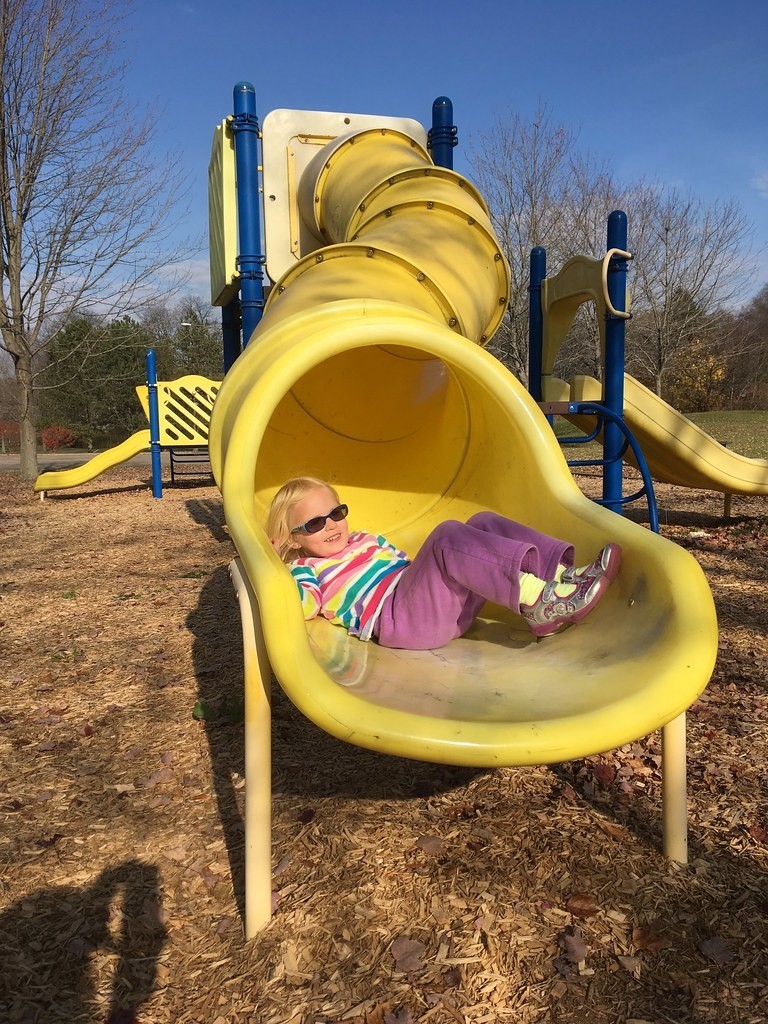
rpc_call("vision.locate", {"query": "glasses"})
[291,503,349,535]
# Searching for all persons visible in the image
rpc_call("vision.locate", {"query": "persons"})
[265,477,626,652]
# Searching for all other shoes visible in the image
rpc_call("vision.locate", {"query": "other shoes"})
[563,542,621,582]
[520,574,610,638]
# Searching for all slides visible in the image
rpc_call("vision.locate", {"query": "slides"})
[206,127,721,768]
[33,427,151,493]
[543,370,768,495]
